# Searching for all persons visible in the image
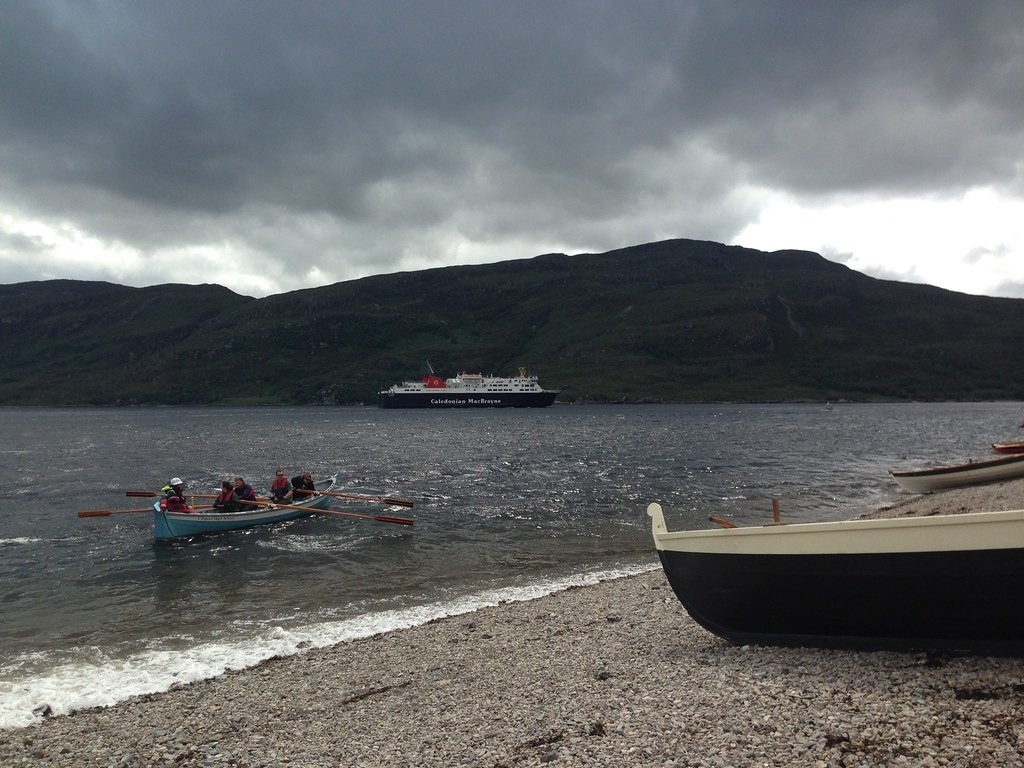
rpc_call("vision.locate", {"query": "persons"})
[269,470,293,505]
[291,472,320,501]
[161,478,190,513]
[233,476,258,512]
[213,481,239,513]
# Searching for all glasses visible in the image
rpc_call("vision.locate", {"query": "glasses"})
[277,474,284,477]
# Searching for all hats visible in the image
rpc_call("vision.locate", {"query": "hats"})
[171,477,184,485]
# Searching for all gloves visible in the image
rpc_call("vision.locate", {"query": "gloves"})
[270,497,278,504]
[314,490,320,496]
[224,503,230,508]
[277,497,285,502]
[213,503,218,508]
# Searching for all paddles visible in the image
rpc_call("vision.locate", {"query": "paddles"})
[126,491,219,499]
[78,509,152,518]
[293,489,414,508]
[239,499,414,526]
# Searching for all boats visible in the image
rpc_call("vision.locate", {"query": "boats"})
[377,360,561,408]
[992,441,1024,455]
[647,502,1024,652]
[887,453,1024,496]
[153,476,336,540]
